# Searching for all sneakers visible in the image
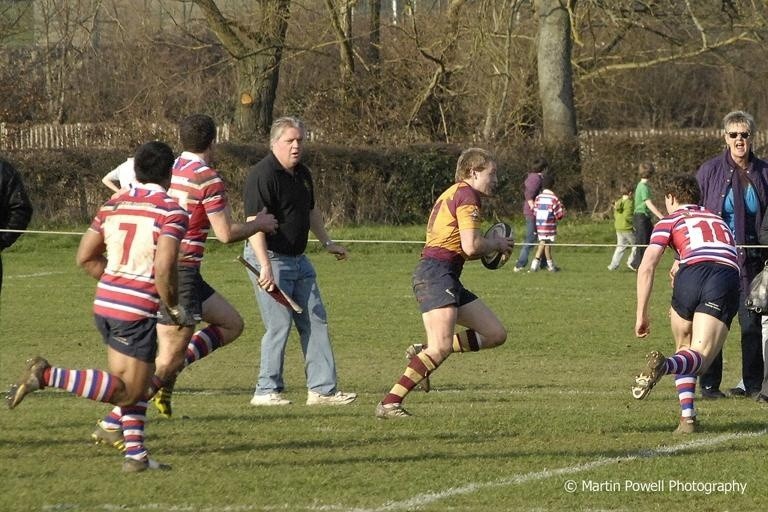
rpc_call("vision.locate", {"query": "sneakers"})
[4,355,50,409]
[305,388,357,406]
[152,380,173,419]
[89,420,128,453]
[249,390,293,406]
[548,265,559,273]
[729,387,746,397]
[512,265,527,273]
[744,390,768,403]
[674,415,697,435]
[607,265,617,271]
[531,259,540,271]
[701,387,726,399]
[628,265,638,272]
[403,342,431,393]
[630,349,667,400]
[374,399,413,417]
[121,455,174,476]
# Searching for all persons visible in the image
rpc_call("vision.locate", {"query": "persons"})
[632,161,666,271]
[242,115,357,406]
[0,152,34,292]
[375,148,514,420]
[5,141,189,473]
[669,110,764,399]
[100,156,143,200]
[514,155,549,270]
[606,183,638,270]
[636,178,740,437]
[90,115,279,453]
[528,176,565,274]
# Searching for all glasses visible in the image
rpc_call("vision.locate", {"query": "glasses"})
[725,131,753,139]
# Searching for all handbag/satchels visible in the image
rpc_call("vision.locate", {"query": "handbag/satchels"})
[745,260,768,316]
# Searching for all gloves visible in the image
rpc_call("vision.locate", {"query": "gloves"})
[166,304,187,328]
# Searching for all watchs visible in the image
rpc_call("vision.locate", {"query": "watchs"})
[321,242,334,247]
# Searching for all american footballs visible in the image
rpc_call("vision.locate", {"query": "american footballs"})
[481,223,514,270]
